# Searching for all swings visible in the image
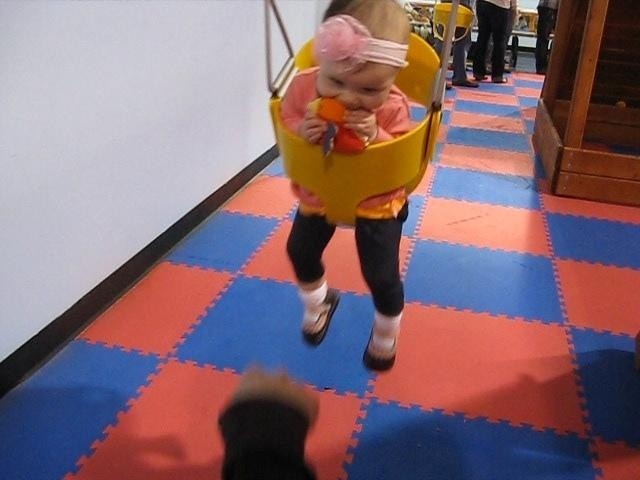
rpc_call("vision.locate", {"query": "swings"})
[264,0,458,226]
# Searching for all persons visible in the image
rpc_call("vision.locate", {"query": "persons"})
[432,0,480,91]
[534,0,559,76]
[473,1,518,84]
[277,0,414,372]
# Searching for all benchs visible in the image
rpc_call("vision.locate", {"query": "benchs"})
[468,25,555,73]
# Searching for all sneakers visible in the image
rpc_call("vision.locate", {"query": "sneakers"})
[302,288,340,344]
[452,78,479,88]
[363,321,400,370]
